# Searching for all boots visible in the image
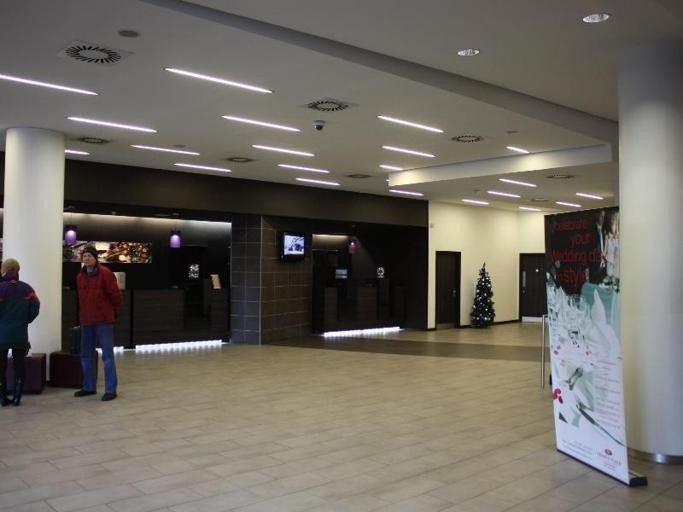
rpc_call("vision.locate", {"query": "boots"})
[0,374,24,406]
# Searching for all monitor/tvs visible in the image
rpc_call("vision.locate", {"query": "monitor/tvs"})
[278,230,306,262]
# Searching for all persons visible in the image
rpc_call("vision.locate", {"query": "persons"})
[288,238,302,252]
[0,257,40,407]
[592,210,620,277]
[76,246,122,401]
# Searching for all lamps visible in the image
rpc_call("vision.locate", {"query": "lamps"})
[64,212,77,245]
[167,227,183,249]
[347,239,358,255]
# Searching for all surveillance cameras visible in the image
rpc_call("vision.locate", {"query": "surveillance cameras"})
[312,120,325,130]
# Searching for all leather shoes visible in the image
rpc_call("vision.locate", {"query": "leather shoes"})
[101,393,117,401]
[74,389,97,398]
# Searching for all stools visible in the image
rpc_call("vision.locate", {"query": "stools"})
[50,350,98,388]
[6,353,46,394]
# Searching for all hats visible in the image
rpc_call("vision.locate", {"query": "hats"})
[80,245,97,260]
